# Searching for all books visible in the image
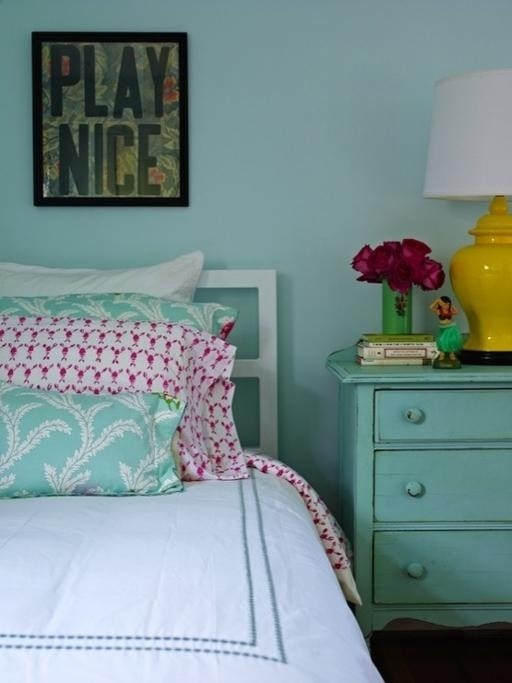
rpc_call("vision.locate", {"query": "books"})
[355,355,424,366]
[356,344,437,358]
[361,333,432,341]
[363,340,436,347]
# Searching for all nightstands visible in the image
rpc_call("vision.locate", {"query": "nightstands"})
[326,333,512,645]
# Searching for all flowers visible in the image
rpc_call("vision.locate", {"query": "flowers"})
[349,238,445,296]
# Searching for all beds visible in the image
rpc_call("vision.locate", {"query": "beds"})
[0,269,386,683]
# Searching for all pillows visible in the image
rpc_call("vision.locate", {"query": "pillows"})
[0,315,250,481]
[0,384,186,499]
[0,293,239,343]
[1,250,203,303]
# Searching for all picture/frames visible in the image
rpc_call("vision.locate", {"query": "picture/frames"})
[31,31,188,207]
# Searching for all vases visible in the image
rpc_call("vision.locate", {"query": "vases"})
[382,276,413,336]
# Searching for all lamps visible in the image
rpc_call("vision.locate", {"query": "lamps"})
[424,68,512,366]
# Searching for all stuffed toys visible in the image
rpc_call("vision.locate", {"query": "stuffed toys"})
[429,295,464,369]
